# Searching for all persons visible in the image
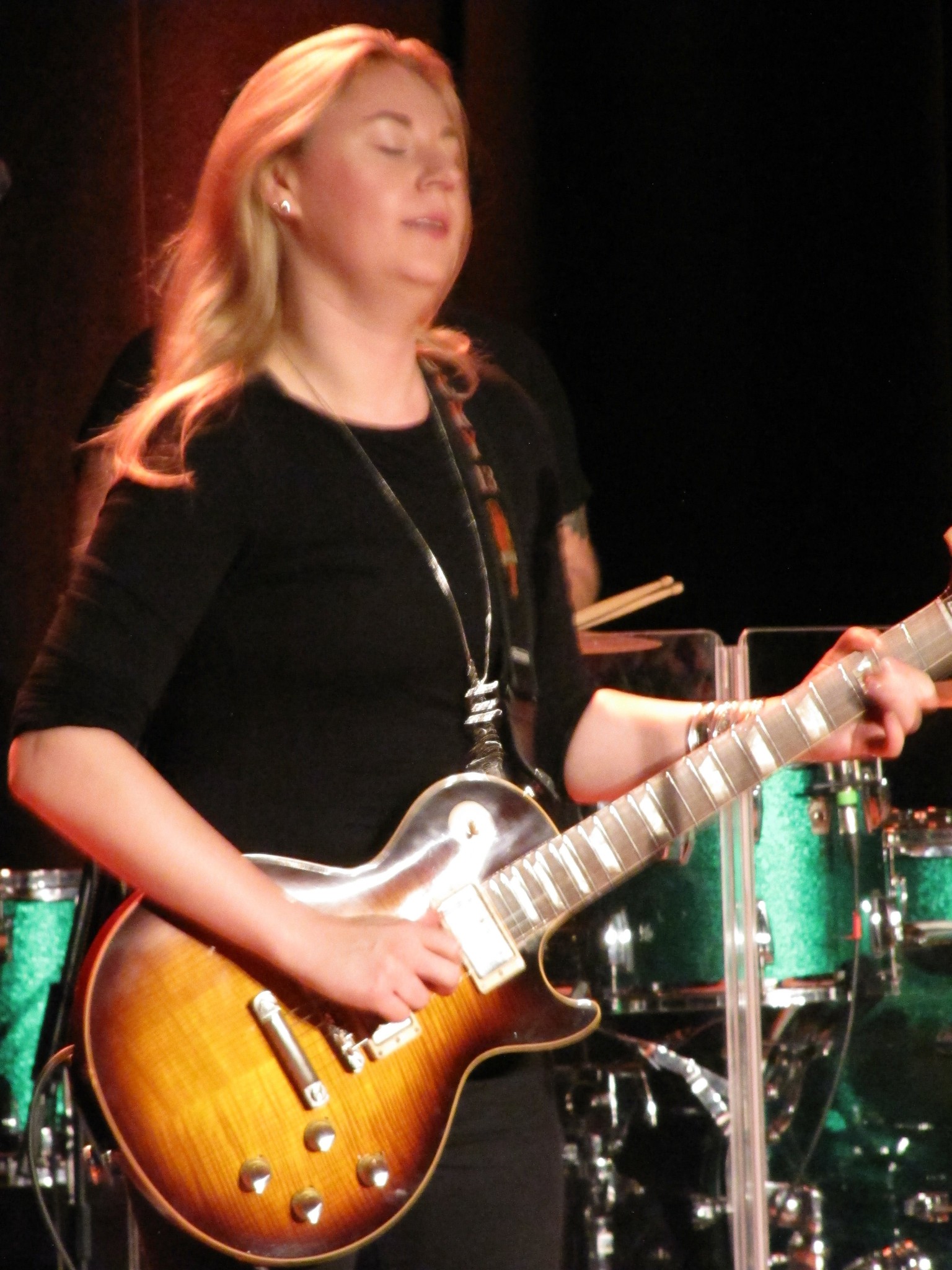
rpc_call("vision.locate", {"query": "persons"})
[8,23,936,1269]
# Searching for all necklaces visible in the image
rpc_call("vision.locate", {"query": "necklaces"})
[274,335,504,777]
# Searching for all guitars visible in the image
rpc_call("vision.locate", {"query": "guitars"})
[63,589,951,1270]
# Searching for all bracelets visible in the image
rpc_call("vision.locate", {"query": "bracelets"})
[690,699,767,754]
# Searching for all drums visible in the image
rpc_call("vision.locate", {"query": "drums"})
[0,867,122,1188]
[572,758,887,1019]
[570,807,951,1270]
